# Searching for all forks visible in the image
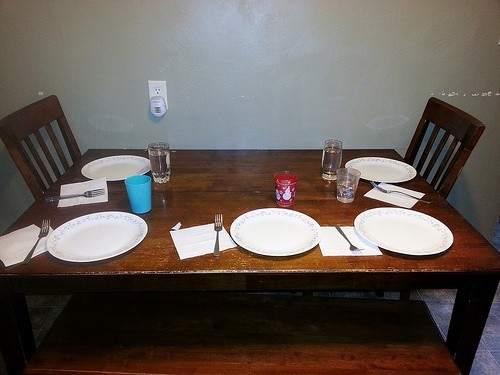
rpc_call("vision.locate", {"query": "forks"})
[213,213,223,257]
[42,188,104,204]
[335,224,364,254]
[371,181,431,206]
[23,219,50,265]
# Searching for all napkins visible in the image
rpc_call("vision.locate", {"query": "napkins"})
[0,224,48,267]
[58,178,109,207]
[363,182,426,209]
[319,226,383,257]
[170,223,238,261]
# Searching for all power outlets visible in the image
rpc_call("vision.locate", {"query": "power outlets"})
[148,80,168,110]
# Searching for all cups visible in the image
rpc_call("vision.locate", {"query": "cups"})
[336,167,361,203]
[148,142,171,183]
[319,140,342,181]
[124,175,153,214]
[272,169,298,209]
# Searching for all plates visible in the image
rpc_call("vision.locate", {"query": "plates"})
[229,208,321,257]
[81,156,151,182]
[46,211,149,263]
[345,157,417,183]
[354,208,454,257]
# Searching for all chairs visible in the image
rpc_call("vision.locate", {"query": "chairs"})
[303,97,486,300]
[0,95,82,201]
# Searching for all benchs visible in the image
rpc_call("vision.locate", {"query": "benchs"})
[24,293,462,375]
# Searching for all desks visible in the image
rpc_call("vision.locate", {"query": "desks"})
[0,148,500,375]
